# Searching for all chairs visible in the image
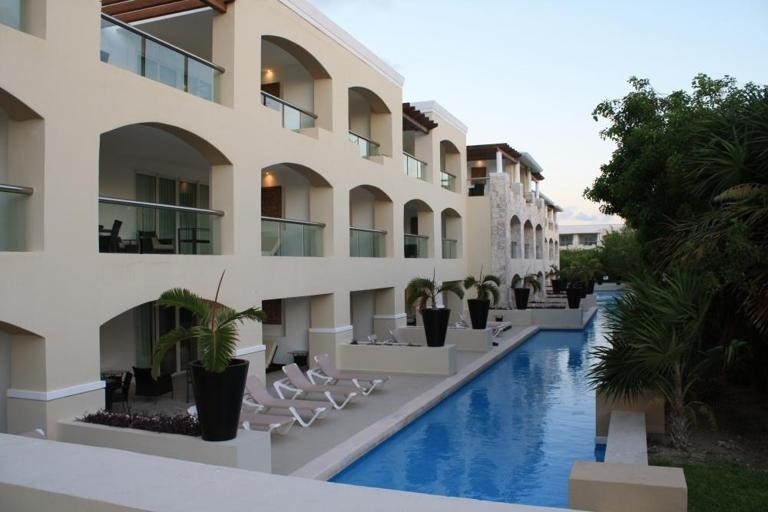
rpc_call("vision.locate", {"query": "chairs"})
[356,329,423,346]
[106,371,132,410]
[455,314,512,338]
[306,353,390,395]
[99,219,174,253]
[132,366,174,404]
[245,375,333,427]
[274,363,366,410]
[187,404,297,436]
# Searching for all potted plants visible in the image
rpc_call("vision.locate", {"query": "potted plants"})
[463,265,500,329]
[510,273,541,309]
[544,265,622,308]
[149,269,268,440]
[405,268,464,347]
